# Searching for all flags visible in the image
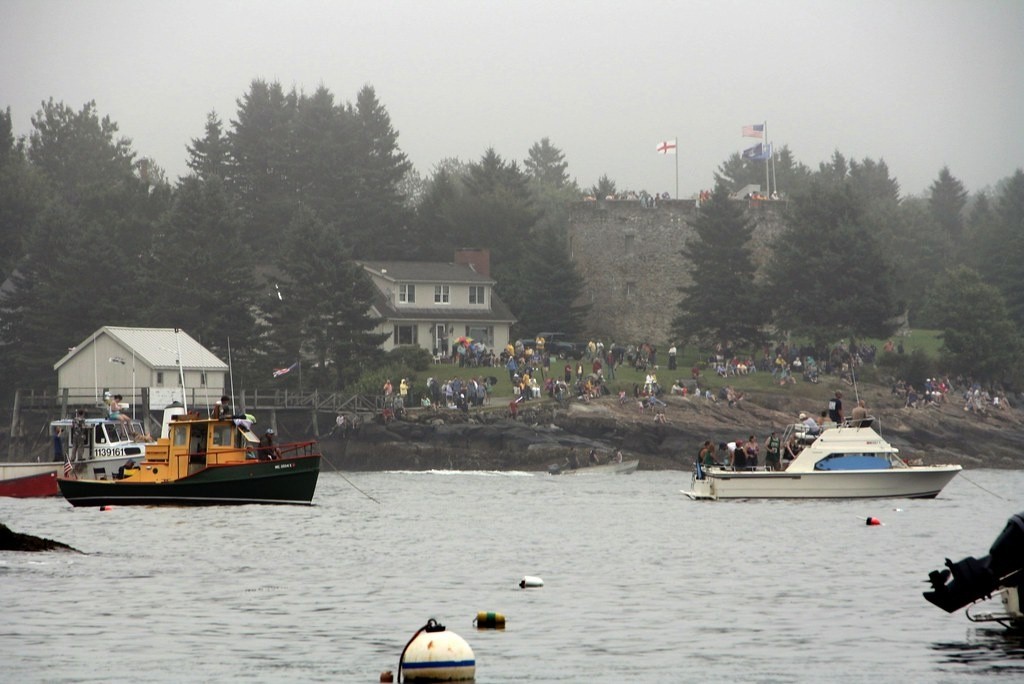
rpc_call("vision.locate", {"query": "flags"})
[742,143,772,160]
[656,139,677,156]
[63,452,72,478]
[273,363,298,380]
[742,124,765,139]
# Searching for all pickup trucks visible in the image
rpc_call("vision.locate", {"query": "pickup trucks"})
[518,332,587,360]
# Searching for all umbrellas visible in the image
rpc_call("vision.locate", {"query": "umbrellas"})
[453,336,476,345]
[484,376,498,386]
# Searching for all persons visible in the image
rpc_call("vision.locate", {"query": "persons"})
[580,188,672,207]
[692,188,717,206]
[108,395,134,436]
[71,409,86,462]
[246,428,283,460]
[52,426,65,462]
[744,190,784,209]
[335,330,1012,480]
[210,395,230,418]
[118,457,136,479]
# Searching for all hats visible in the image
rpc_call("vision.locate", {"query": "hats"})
[736,440,743,447]
[718,442,726,450]
[799,413,806,420]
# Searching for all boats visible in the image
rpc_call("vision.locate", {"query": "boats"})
[0,418,156,498]
[548,458,640,475]
[679,360,965,502]
[923,510,1024,643]
[48,326,322,508]
